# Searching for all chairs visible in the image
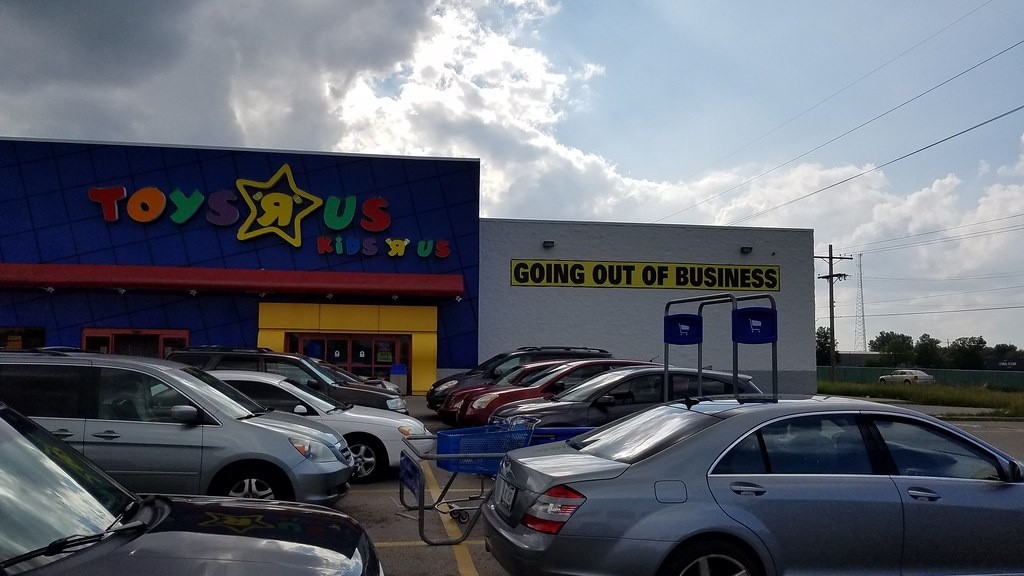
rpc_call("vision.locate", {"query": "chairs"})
[123,380,149,420]
[830,426,862,450]
[782,421,833,455]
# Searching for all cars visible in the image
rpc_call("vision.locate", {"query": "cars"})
[878,369,936,386]
[0,403,386,576]
[151,370,436,480]
[480,393,1024,575]
[274,358,402,394]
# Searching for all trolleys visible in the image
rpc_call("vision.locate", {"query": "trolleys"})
[436,416,543,521]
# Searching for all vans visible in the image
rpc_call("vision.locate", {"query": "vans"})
[0,348,355,508]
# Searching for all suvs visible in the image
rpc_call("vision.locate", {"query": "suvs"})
[486,368,765,445]
[424,344,674,426]
[163,347,411,416]
